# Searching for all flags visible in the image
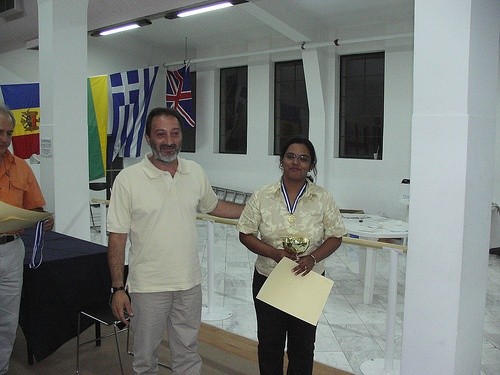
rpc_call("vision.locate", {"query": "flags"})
[111,66,159,161]
[0,83,41,159]
[166,63,195,130]
[89,76,109,180]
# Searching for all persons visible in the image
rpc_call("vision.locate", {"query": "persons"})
[107,108,246,375]
[0,108,54,375]
[235,137,347,375]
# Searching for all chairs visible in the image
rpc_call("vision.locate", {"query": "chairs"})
[76,265,173,375]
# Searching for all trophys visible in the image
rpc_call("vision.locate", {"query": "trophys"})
[283,237,310,263]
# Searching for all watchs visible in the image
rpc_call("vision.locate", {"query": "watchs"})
[110,286,125,294]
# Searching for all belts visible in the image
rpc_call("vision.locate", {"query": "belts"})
[0,235,19,245]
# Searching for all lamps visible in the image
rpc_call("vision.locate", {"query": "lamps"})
[91,19,152,37]
[164,0,249,20]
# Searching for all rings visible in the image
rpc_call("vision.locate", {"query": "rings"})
[305,265,307,270]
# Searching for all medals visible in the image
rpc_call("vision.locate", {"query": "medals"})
[288,214,295,224]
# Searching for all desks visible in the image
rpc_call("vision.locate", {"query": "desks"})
[18,227,113,366]
[339,213,408,304]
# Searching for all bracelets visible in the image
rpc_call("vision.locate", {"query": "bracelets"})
[310,254,317,266]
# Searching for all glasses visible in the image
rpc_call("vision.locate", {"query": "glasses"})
[284,153,311,162]
